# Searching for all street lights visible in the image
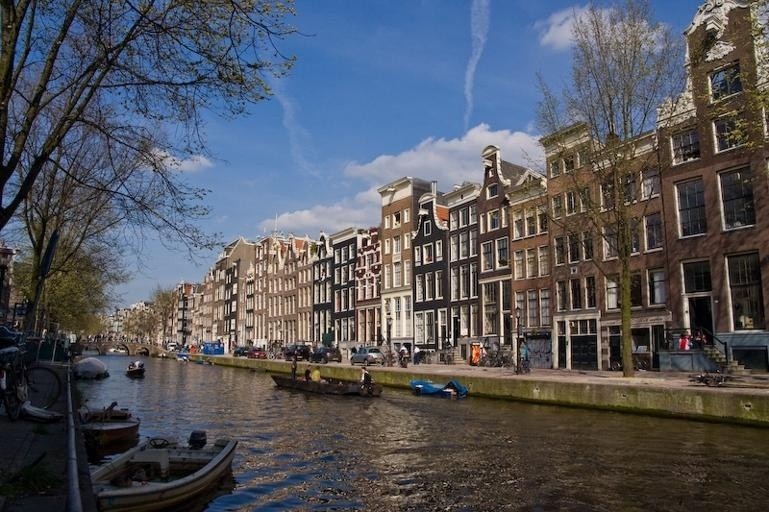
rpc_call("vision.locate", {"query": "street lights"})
[515,307,522,374]
[386,314,392,367]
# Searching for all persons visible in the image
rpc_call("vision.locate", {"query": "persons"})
[128,359,145,370]
[94,334,158,345]
[351,346,356,354]
[312,366,321,382]
[291,356,296,380]
[362,367,367,383]
[305,364,312,381]
[695,332,707,345]
[679,334,693,351]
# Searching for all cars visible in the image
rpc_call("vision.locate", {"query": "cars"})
[233,347,248,357]
[246,347,266,359]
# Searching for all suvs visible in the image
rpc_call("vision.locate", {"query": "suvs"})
[350,346,384,366]
[308,346,341,365]
[285,344,312,362]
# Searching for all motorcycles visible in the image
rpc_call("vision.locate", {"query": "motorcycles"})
[399,355,408,368]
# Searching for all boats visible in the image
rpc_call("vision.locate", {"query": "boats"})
[88,401,140,443]
[157,352,216,367]
[88,428,237,512]
[409,378,472,399]
[269,373,382,397]
[125,366,144,377]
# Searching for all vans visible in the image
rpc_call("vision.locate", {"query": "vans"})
[166,343,177,352]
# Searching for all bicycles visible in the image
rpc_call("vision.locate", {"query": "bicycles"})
[610,356,649,371]
[478,349,530,374]
[0,325,62,421]
